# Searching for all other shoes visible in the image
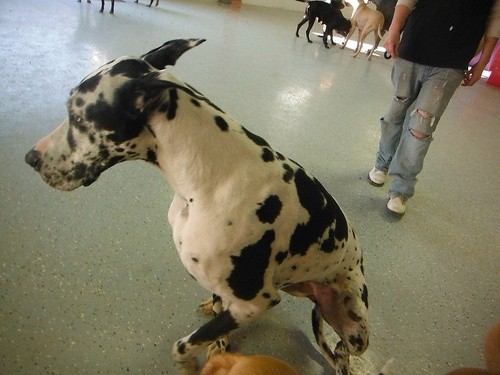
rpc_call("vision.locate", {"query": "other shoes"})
[368,167,386,186]
[385,197,406,216]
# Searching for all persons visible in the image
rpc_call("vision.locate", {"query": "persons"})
[369,0,500,214]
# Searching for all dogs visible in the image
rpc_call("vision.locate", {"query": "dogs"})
[26,37,381,375]
[295,0,385,60]
